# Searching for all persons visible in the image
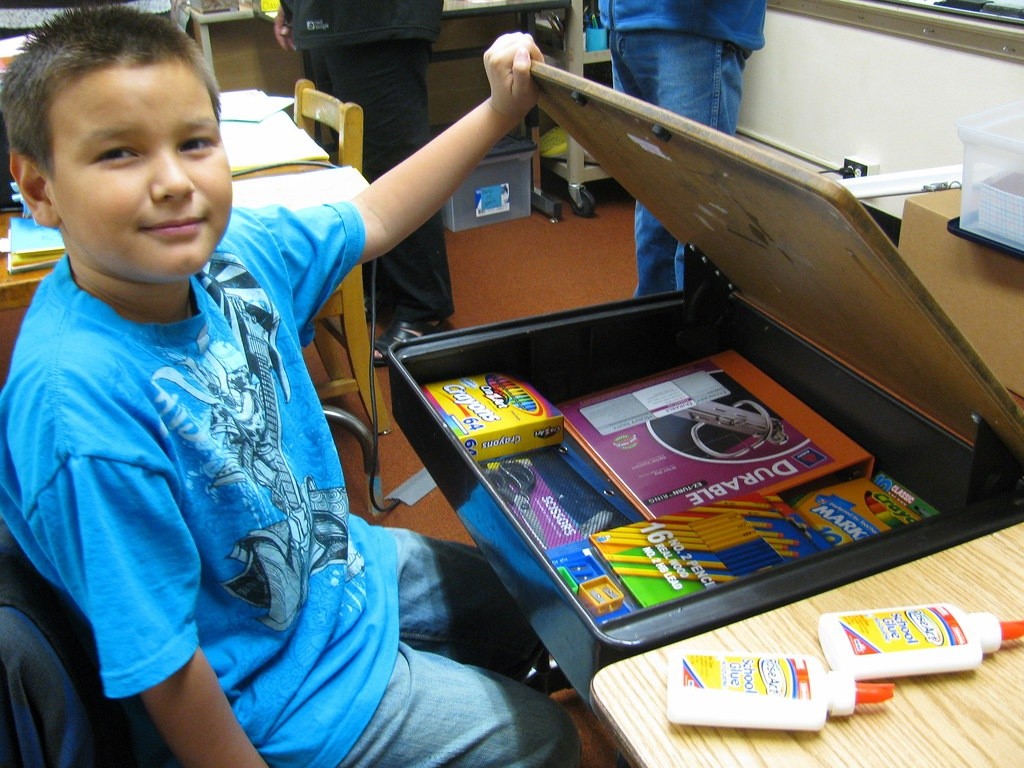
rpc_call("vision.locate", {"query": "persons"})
[0,4,582,768]
[275,0,456,367]
[599,0,768,297]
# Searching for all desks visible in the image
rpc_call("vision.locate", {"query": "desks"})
[0,163,349,391]
[187,5,563,224]
[587,520,1024,766]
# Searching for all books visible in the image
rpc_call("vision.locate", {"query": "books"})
[6,217,68,275]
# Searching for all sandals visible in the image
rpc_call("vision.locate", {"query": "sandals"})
[361,289,395,321]
[373,315,450,367]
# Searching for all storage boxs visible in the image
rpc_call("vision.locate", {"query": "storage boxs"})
[388,60,1024,704]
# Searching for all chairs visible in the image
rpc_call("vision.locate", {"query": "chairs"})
[1,554,184,768]
[294,78,392,436]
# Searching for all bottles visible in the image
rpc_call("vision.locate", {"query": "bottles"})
[665,649,897,733]
[816,603,1023,681]
